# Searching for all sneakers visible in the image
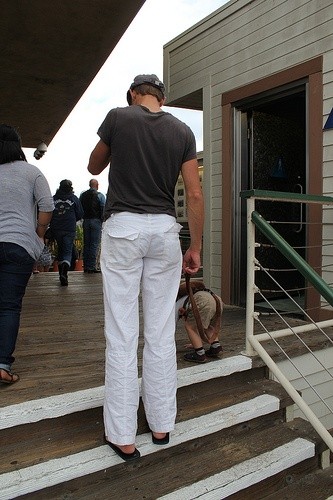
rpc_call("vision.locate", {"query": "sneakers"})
[84,268,92,272]
[206,346,223,356]
[182,351,207,362]
[88,269,101,274]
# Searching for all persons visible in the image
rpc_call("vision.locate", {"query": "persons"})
[0,124,55,385]
[47,179,83,287]
[174,281,223,364]
[88,74,205,463]
[38,241,54,273]
[79,179,107,273]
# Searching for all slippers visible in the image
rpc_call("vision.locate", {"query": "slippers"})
[152,431,169,444]
[104,432,140,461]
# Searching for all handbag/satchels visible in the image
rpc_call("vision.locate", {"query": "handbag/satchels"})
[44,228,55,246]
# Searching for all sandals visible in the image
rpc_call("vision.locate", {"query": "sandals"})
[0,369,19,386]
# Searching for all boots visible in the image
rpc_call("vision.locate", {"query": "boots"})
[60,262,68,286]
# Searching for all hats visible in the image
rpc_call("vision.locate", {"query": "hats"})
[127,74,165,106]
